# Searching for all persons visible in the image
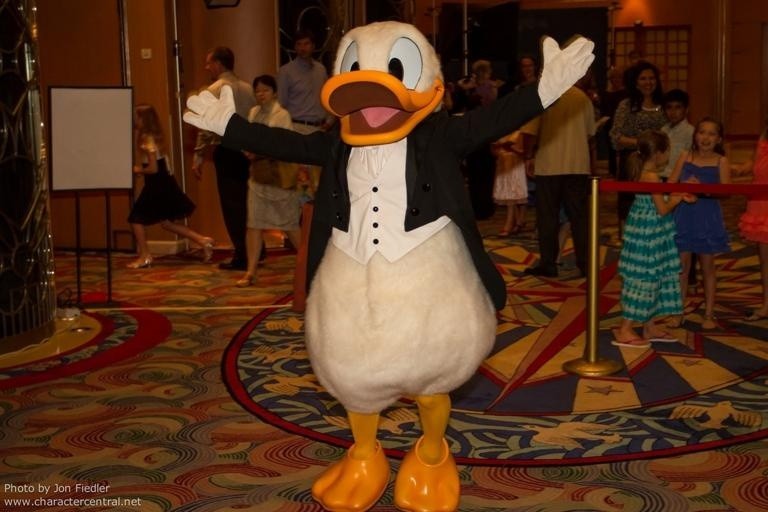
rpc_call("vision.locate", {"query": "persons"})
[188,29,324,287]
[445,51,767,349]
[181,21,596,512]
[127,103,215,270]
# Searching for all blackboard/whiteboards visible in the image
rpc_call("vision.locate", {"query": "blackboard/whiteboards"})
[48,85,136,194]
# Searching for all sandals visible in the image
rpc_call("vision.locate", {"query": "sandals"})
[744,311,767,321]
[127,255,152,269]
[204,236,213,262]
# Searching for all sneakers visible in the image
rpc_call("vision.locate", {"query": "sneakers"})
[666,317,681,327]
[701,316,715,331]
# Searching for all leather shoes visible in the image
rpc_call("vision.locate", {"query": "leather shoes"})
[237,274,256,286]
[525,267,558,277]
[219,262,247,271]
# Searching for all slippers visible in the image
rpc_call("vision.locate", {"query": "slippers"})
[648,332,679,342]
[612,338,652,349]
